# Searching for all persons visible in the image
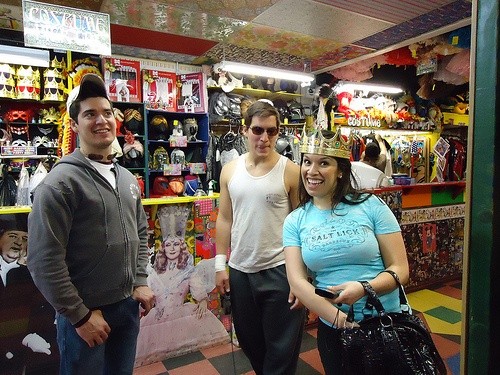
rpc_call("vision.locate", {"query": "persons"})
[215,101,306,375]
[350,142,390,190]
[283,122,410,375]
[27,73,156,375]
[360,134,387,171]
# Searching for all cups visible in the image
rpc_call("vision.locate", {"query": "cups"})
[395,176,411,185]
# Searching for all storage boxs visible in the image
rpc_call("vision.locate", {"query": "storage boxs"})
[102,56,206,112]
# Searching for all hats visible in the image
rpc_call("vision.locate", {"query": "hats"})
[66,74,110,119]
[157,206,190,241]
[365,143,379,157]
[0,215,28,233]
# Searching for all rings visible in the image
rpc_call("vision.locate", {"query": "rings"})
[337,303,342,306]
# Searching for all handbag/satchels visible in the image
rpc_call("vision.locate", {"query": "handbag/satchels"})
[339,269,447,375]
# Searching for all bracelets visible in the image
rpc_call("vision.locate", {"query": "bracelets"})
[215,254,227,273]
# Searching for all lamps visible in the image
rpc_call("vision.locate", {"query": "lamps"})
[0,45,50,67]
[340,79,403,94]
[214,60,314,83]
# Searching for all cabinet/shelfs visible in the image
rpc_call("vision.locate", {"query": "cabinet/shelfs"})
[0,77,310,214]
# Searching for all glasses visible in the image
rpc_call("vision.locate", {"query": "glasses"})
[247,126,279,136]
[81,146,118,160]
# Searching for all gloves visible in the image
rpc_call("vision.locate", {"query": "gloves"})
[21,333,51,356]
